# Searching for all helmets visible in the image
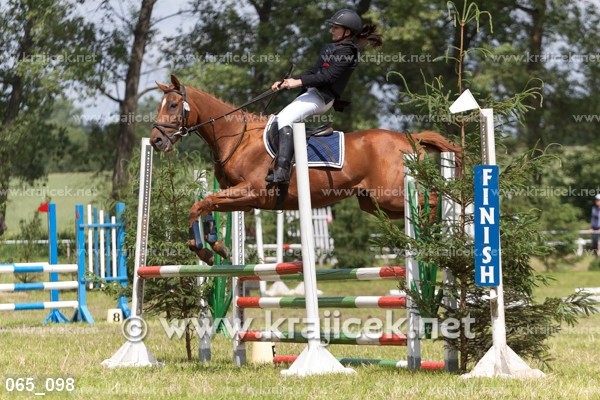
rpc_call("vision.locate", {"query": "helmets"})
[326,10,363,42]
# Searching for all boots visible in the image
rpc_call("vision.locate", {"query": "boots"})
[266,126,295,186]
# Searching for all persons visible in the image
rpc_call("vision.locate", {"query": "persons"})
[265,8,383,186]
[589,193,600,229]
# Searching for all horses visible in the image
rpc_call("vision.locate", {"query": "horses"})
[149,73,468,267]
